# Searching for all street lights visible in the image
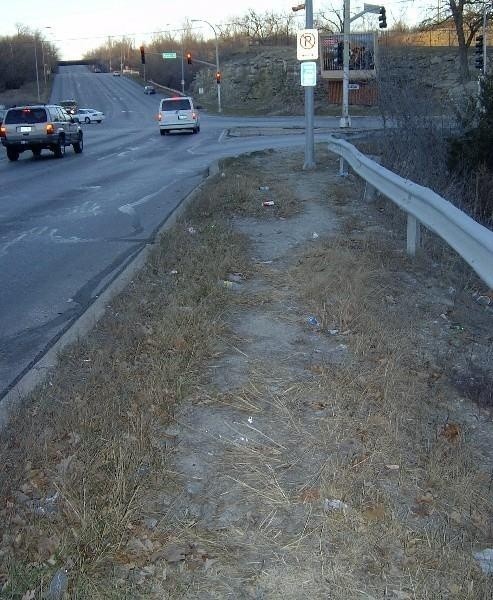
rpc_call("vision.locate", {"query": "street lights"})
[34,26,52,98]
[191,19,223,112]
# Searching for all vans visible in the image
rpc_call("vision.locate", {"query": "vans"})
[159,97,200,134]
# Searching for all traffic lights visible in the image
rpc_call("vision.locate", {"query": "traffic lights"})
[378,8,387,28]
[216,72,220,83]
[187,53,192,64]
[475,35,484,68]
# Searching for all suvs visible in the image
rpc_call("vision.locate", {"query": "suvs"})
[1,104,83,158]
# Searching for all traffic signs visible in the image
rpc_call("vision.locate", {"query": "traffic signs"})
[163,53,176,59]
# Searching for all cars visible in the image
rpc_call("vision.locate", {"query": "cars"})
[144,86,154,94]
[60,99,105,123]
[113,71,120,76]
